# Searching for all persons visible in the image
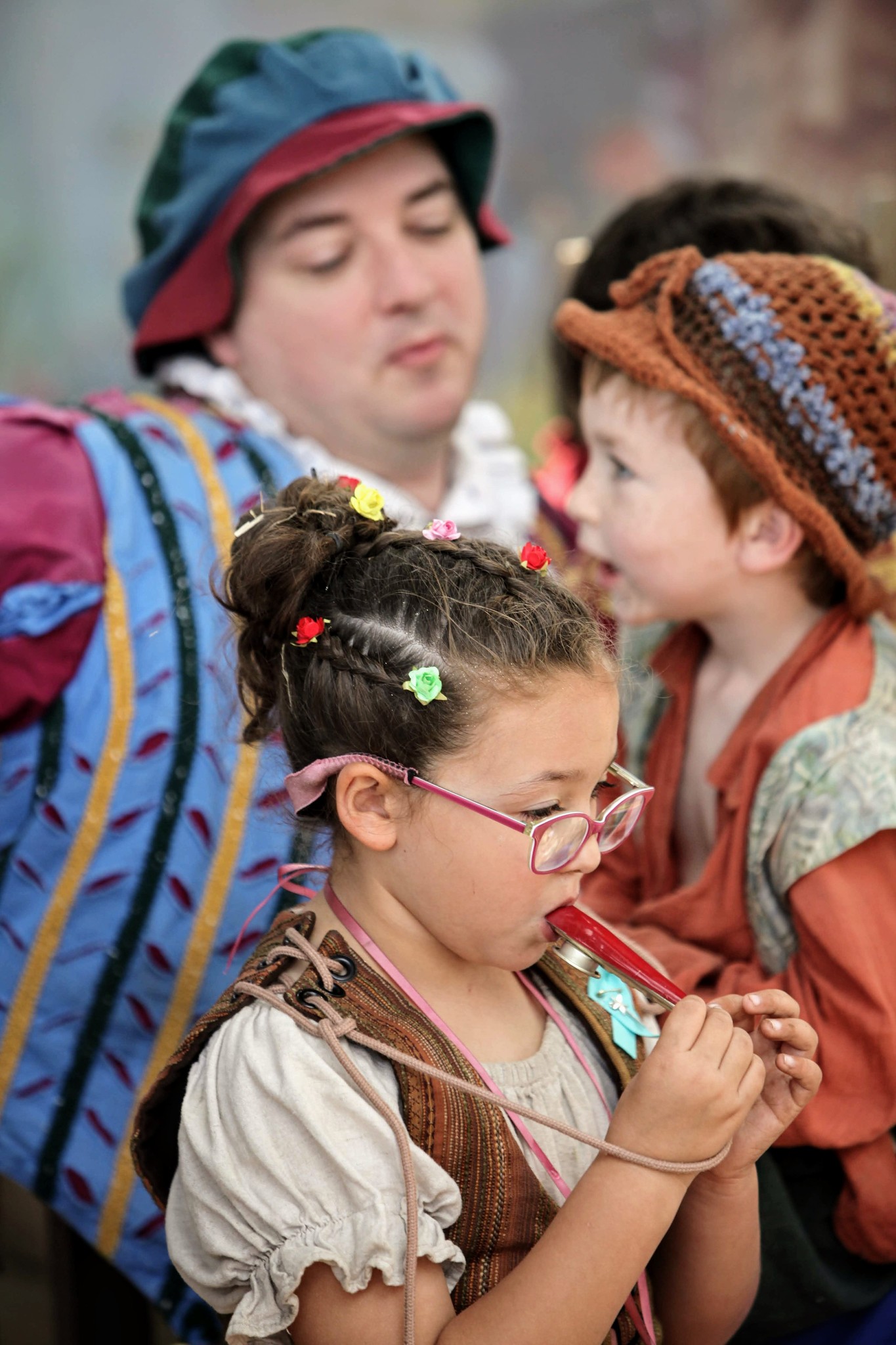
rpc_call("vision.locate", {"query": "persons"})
[0,26,571,1345]
[131,477,824,1345]
[533,174,895,1345]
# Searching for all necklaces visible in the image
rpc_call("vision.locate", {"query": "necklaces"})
[224,862,655,1345]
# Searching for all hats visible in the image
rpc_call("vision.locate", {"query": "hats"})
[119,26,512,379]
[552,244,896,627]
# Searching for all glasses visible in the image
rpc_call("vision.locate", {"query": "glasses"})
[305,754,655,874]
